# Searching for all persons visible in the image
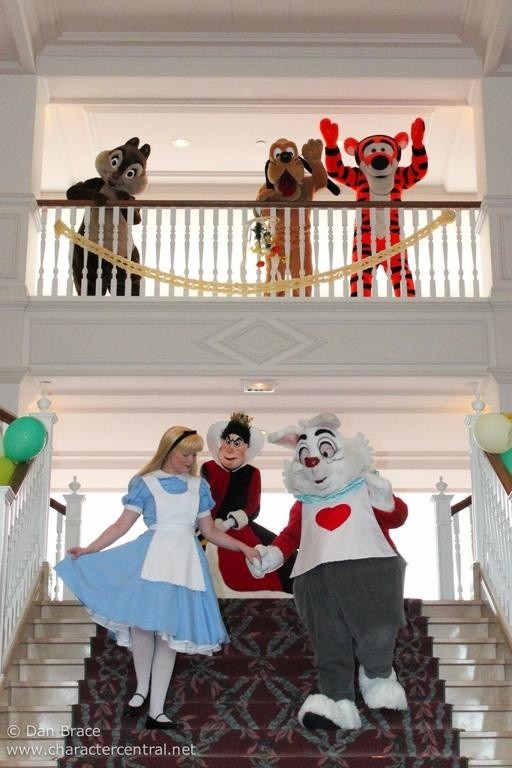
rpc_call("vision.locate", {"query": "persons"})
[52,423,263,730]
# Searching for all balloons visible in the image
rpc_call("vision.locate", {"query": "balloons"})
[1,415,50,464]
[472,412,511,455]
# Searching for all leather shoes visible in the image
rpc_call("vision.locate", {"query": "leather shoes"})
[145,714,184,729]
[126,692,149,717]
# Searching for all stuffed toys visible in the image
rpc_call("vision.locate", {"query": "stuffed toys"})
[188,410,302,598]
[66,136,152,296]
[253,137,327,298]
[245,412,412,729]
[320,115,428,297]
